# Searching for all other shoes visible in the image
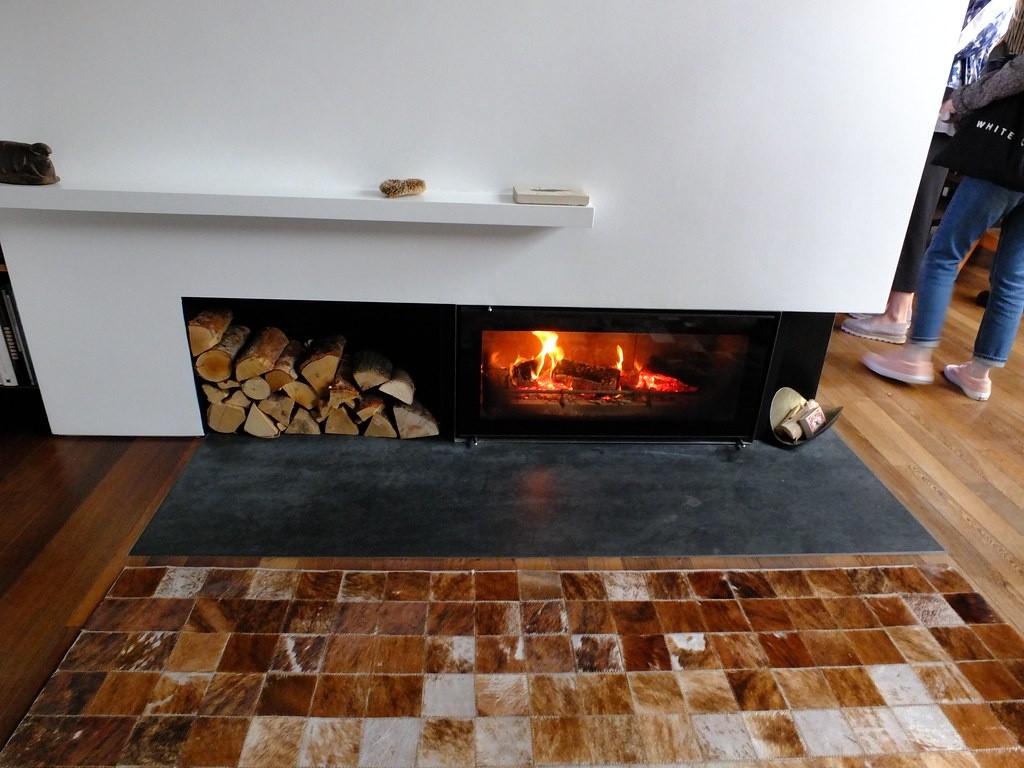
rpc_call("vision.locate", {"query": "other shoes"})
[842,313,911,343]
[861,350,934,384]
[945,364,992,401]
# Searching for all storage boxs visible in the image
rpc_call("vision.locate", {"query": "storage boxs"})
[799,406,826,439]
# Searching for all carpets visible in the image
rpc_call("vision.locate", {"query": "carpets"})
[127,425,947,558]
[1,562,1024,768]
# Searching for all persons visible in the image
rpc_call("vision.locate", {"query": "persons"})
[859,0,1024,400]
[840,0,1015,344]
